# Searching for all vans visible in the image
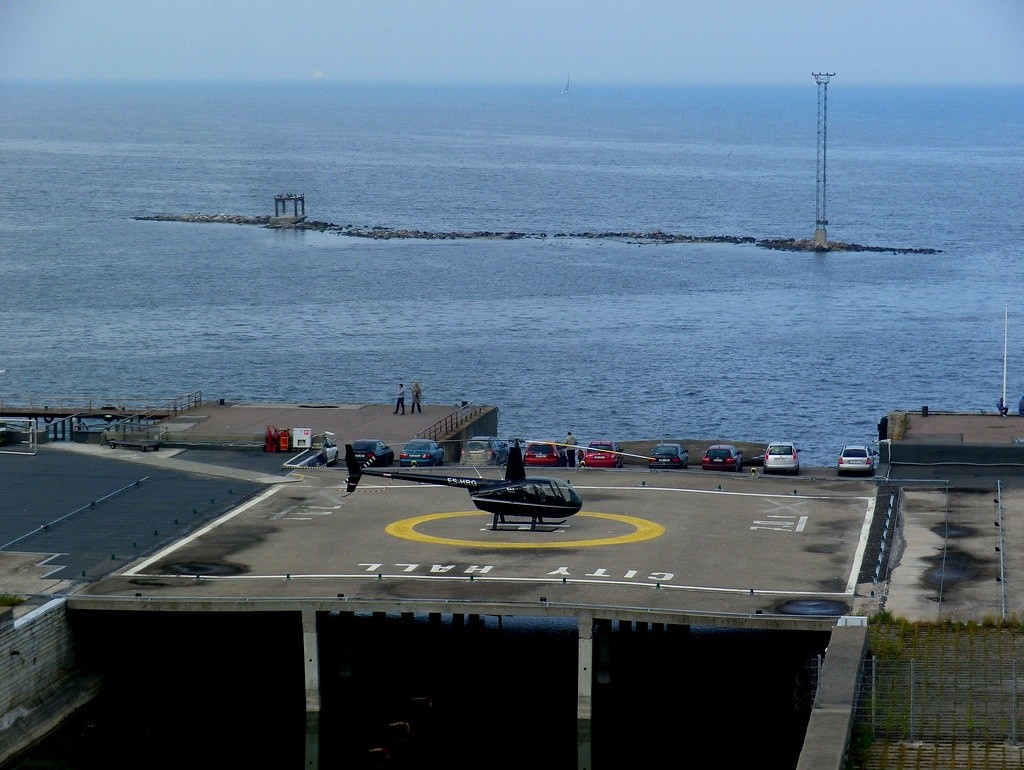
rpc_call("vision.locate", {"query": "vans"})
[762,441,802,476]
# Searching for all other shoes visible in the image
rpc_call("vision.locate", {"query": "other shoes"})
[392,411,396,414]
[400,413,404,415]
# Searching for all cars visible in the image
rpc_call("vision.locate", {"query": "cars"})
[701,444,743,472]
[460,435,508,466]
[326,437,339,466]
[649,443,688,469]
[351,439,395,468]
[523,438,567,467]
[584,440,624,468]
[400,439,445,467]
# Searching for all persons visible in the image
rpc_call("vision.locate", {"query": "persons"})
[410,383,423,414]
[998,396,1024,417]
[392,384,405,415]
[564,432,578,467]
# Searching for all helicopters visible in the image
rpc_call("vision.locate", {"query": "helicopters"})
[345,436,657,532]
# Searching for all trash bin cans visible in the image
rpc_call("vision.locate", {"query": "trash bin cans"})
[922,406,928,417]
[220,398,225,405]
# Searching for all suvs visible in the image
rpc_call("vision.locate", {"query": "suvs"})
[837,445,875,477]
